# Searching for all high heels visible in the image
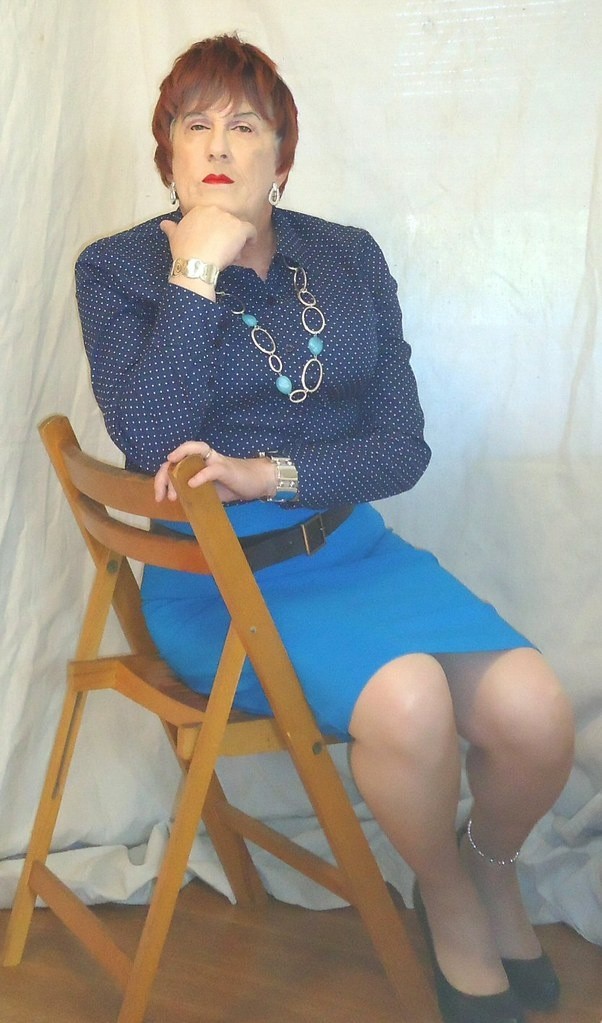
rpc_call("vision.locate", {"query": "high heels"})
[412,877,522,1023]
[455,828,559,1009]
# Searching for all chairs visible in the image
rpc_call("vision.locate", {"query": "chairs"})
[0,415,445,1023]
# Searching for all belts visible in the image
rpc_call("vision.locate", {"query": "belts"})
[150,503,355,573]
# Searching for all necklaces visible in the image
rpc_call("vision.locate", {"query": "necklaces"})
[216,253,326,403]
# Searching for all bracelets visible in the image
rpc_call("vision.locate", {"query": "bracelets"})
[261,452,298,503]
[168,257,219,285]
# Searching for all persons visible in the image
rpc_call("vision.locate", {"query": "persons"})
[74,34,574,1023]
